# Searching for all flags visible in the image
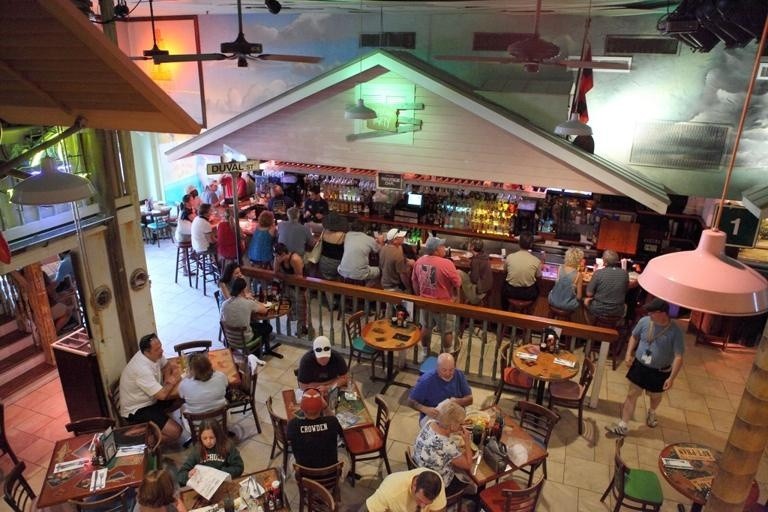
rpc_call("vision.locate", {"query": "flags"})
[571,44,593,126]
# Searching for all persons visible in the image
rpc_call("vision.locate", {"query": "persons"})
[43,271,72,333]
[407,352,473,429]
[175,172,629,344]
[177,355,236,448]
[357,467,448,512]
[410,398,486,512]
[296,336,348,394]
[176,418,244,486]
[137,470,186,512]
[119,333,183,449]
[53,250,76,293]
[605,297,685,437]
[287,389,350,493]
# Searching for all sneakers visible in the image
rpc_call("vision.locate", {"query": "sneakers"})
[647,409,657,428]
[294,325,308,336]
[605,423,627,436]
[183,432,193,447]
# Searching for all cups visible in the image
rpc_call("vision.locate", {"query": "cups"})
[472,425,482,445]
[222,497,234,512]
[247,500,258,512]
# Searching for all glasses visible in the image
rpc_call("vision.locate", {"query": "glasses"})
[315,347,330,352]
[303,388,320,393]
[147,333,155,342]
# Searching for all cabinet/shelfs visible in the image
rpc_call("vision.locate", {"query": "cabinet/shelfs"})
[686,309,733,352]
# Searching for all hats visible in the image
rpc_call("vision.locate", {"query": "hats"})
[425,237,446,249]
[185,185,197,194]
[208,180,219,186]
[387,228,407,240]
[313,336,331,358]
[300,389,322,414]
[643,299,669,312]
[322,212,347,231]
[230,278,246,296]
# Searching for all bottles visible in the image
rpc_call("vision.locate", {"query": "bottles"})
[547,335,555,353]
[484,421,490,444]
[491,414,504,442]
[540,328,546,351]
[263,498,268,511]
[556,342,560,355]
[276,499,281,510]
[268,499,276,510]
[270,481,281,496]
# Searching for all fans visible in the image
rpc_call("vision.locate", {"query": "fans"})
[127,0,168,65]
[155,0,323,67]
[433,0,636,74]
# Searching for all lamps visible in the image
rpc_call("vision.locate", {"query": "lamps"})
[554,0,592,137]
[637,17,768,316]
[9,127,96,204]
[344,0,378,119]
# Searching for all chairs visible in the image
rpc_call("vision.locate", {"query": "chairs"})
[138,202,179,247]
[599,436,664,512]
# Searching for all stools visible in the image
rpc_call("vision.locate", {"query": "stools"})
[193,250,221,296]
[174,241,206,286]
[218,256,238,280]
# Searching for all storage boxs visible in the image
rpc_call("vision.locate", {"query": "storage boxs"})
[710,195,763,249]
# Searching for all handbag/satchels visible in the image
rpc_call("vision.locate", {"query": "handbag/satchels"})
[307,229,325,264]
[483,438,509,474]
